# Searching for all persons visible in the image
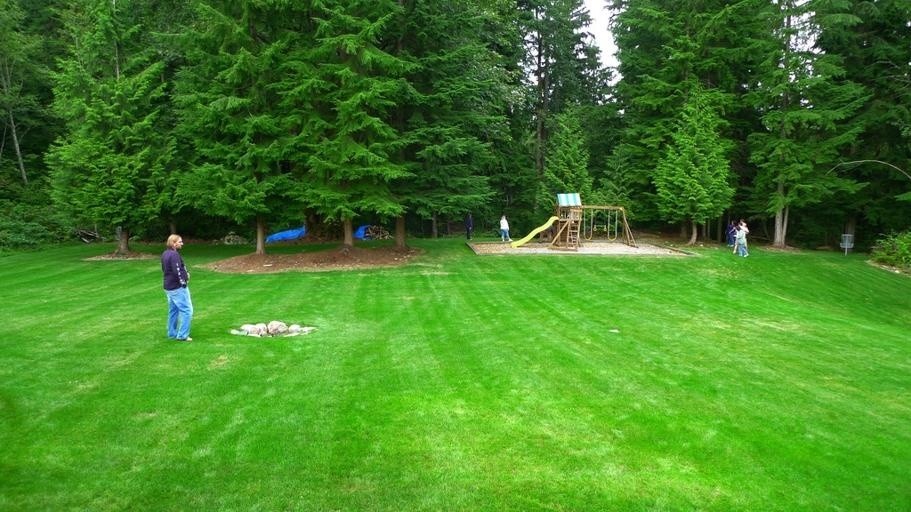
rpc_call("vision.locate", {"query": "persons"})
[465,214,473,240]
[365,224,393,240]
[725,219,749,257]
[500,216,513,241]
[160,233,193,341]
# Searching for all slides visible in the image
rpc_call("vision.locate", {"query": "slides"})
[510,216,559,249]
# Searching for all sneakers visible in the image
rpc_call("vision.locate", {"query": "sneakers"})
[502,238,513,242]
[185,337,193,341]
[727,244,749,257]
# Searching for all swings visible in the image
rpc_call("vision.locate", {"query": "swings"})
[584,209,593,240]
[594,209,607,231]
[608,209,617,241]
